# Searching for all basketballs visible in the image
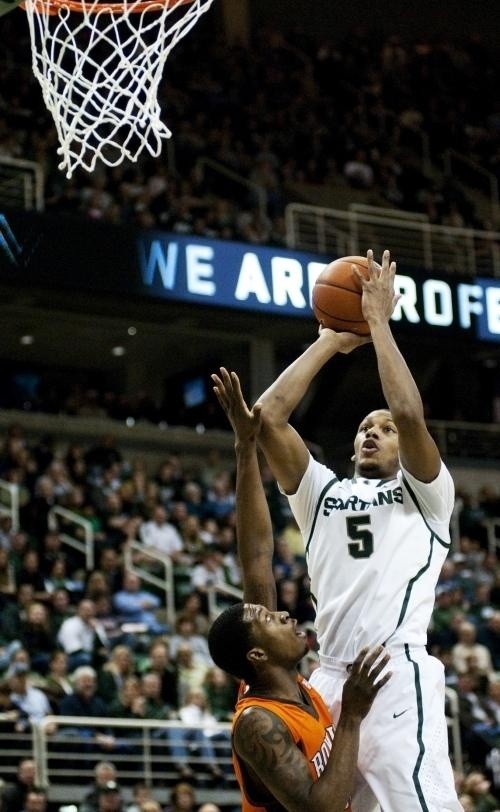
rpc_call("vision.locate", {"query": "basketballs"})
[313,256,382,335]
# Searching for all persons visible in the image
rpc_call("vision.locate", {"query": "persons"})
[255,250,465,812]
[428,485,499,812]
[0,433,319,811]
[210,366,393,812]
[3,15,497,250]
[2,377,498,460]
